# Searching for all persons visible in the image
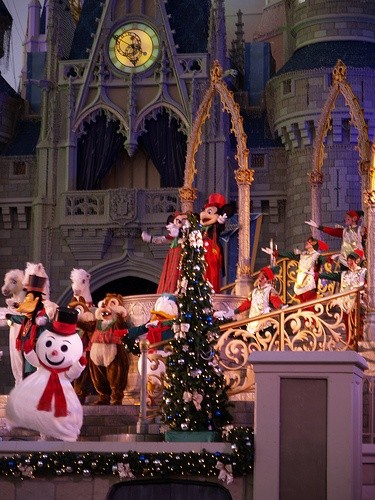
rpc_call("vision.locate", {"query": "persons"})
[303,249,368,351]
[225,264,288,340]
[260,236,339,328]
[304,208,368,271]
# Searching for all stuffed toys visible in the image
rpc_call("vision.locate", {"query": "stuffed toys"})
[141,210,200,294]
[122,292,179,403]
[0,264,23,389]
[76,293,134,406]
[1,275,49,380]
[21,261,55,318]
[198,193,237,293]
[62,296,94,405]
[69,268,98,312]
[4,306,87,443]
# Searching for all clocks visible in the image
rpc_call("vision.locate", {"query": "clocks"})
[104,17,164,79]
